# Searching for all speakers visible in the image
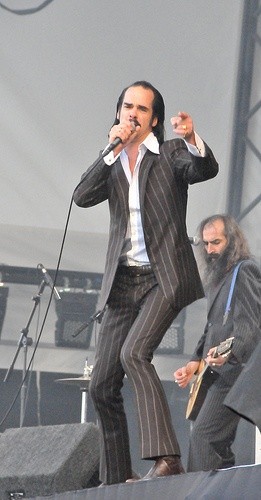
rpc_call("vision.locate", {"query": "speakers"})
[0,423,142,500]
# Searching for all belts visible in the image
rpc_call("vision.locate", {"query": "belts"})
[116,265,153,276]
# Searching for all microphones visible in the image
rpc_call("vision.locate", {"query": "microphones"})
[187,237,201,246]
[103,137,122,158]
[39,264,62,301]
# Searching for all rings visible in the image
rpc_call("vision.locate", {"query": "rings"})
[175,380,177,382]
[212,363,215,366]
[119,128,122,132]
[182,125,186,130]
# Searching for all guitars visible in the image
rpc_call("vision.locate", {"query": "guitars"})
[186,335,236,422]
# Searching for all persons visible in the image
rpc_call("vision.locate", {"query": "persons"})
[73,80,219,487]
[174,215,261,471]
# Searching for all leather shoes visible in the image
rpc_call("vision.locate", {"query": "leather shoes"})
[126,455,185,482]
[98,482,106,487]
